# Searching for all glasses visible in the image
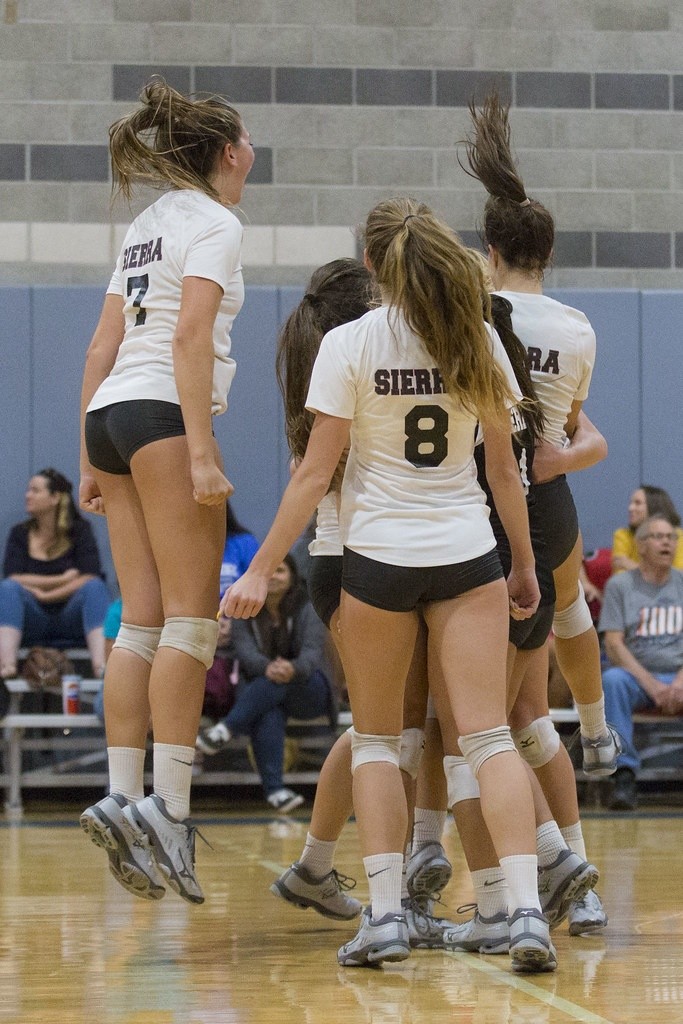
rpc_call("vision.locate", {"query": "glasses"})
[640,531,679,541]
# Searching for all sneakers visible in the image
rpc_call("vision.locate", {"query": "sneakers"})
[336,905,411,965]
[507,907,556,972]
[537,849,599,932]
[400,840,452,914]
[442,904,511,955]
[121,794,213,906]
[567,723,628,776]
[269,863,362,921]
[79,793,166,901]
[359,895,461,952]
[569,886,610,935]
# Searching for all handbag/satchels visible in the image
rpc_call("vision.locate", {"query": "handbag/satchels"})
[21,647,75,687]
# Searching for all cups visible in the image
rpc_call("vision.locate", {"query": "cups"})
[61,675,80,713]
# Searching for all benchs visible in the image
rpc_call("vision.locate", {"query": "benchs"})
[0,646,683,825]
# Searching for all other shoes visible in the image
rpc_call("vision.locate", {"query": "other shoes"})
[0,662,19,680]
[266,815,302,839]
[268,787,304,812]
[195,727,229,756]
[606,768,638,812]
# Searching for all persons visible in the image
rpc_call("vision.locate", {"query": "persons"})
[273,84,608,953]
[76,73,253,905]
[212,196,557,974]
[0,469,683,812]
